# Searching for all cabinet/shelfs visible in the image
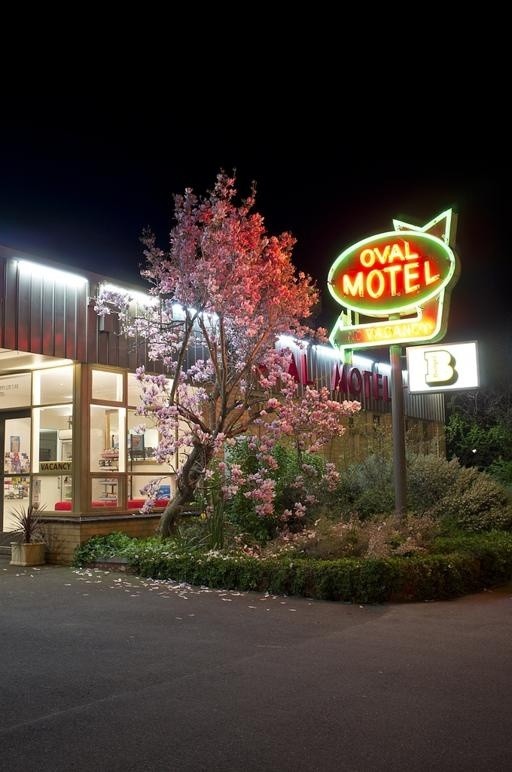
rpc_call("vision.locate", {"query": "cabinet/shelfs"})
[57,428,103,504]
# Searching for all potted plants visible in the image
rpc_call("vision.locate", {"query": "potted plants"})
[6,500,50,568]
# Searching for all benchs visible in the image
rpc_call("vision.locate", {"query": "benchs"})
[54,498,171,511]
